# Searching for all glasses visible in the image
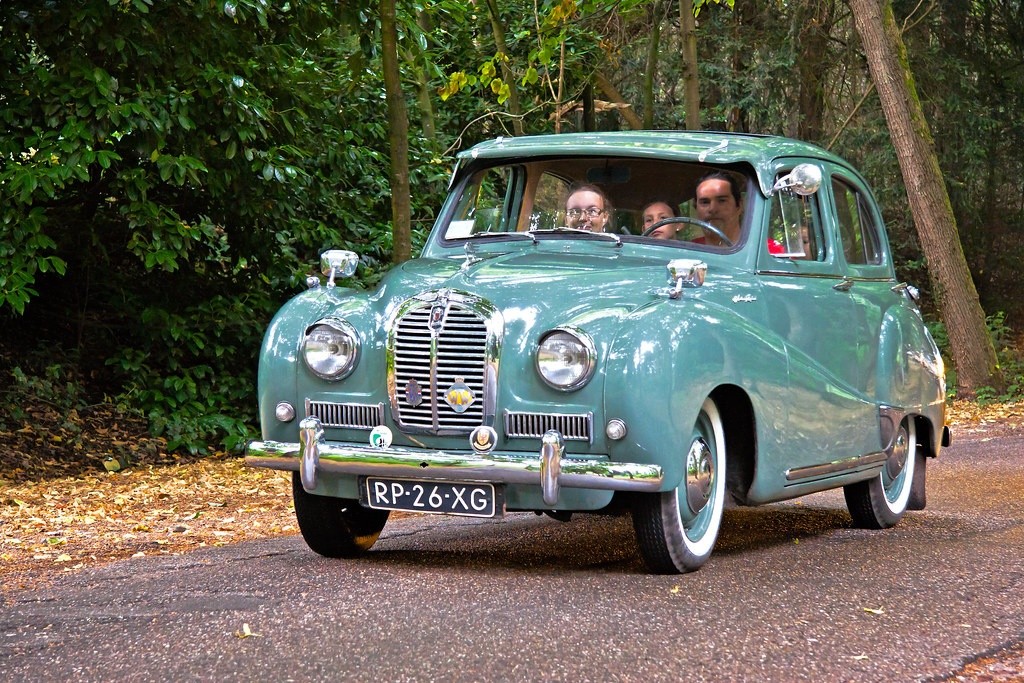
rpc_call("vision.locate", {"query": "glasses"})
[565,208,612,221]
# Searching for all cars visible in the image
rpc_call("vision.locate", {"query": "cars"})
[245,129,953,574]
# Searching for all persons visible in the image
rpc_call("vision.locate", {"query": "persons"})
[793,227,814,259]
[688,172,798,260]
[641,199,686,240]
[564,185,609,232]
